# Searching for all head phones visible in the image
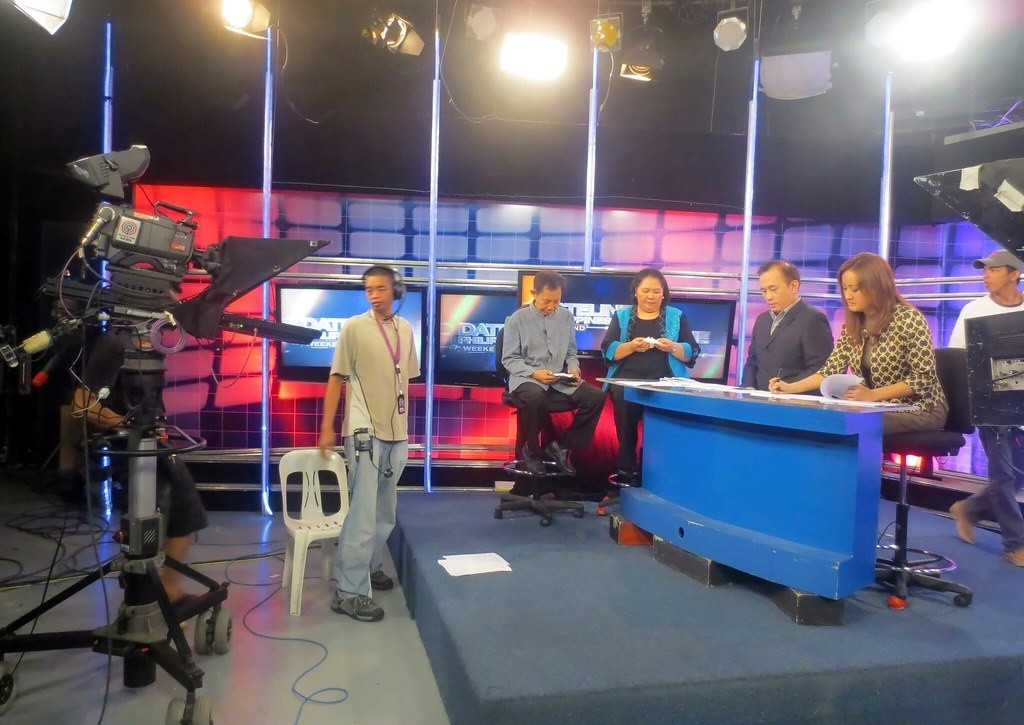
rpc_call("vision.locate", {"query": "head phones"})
[363,266,406,301]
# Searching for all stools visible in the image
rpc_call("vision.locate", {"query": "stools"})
[875,347,975,611]
[493,328,586,527]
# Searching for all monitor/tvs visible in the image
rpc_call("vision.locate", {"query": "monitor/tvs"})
[275,270,737,387]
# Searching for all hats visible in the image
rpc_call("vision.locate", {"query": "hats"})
[972,249,1021,272]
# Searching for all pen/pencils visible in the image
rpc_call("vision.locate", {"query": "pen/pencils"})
[776,368,782,382]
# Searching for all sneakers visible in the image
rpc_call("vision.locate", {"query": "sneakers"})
[330,588,384,622]
[370,571,393,590]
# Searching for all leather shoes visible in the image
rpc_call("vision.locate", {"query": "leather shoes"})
[949,500,976,543]
[617,468,638,482]
[545,439,577,476]
[522,440,547,475]
[1002,548,1024,567]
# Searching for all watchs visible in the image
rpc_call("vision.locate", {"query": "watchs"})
[671,344,677,355]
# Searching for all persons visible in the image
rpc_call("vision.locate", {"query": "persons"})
[600,268,702,486]
[319,265,422,622]
[500,270,606,477]
[741,260,834,395]
[766,251,950,439]
[947,248,1024,568]
[72,330,211,628]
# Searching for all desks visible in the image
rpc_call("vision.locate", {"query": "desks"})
[595,376,921,627]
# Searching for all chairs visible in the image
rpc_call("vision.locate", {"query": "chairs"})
[278,447,373,618]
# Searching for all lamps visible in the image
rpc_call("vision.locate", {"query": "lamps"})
[361,11,427,58]
[5,0,74,36]
[587,15,624,55]
[221,0,272,34]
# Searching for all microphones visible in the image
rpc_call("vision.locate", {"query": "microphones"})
[382,298,404,320]
